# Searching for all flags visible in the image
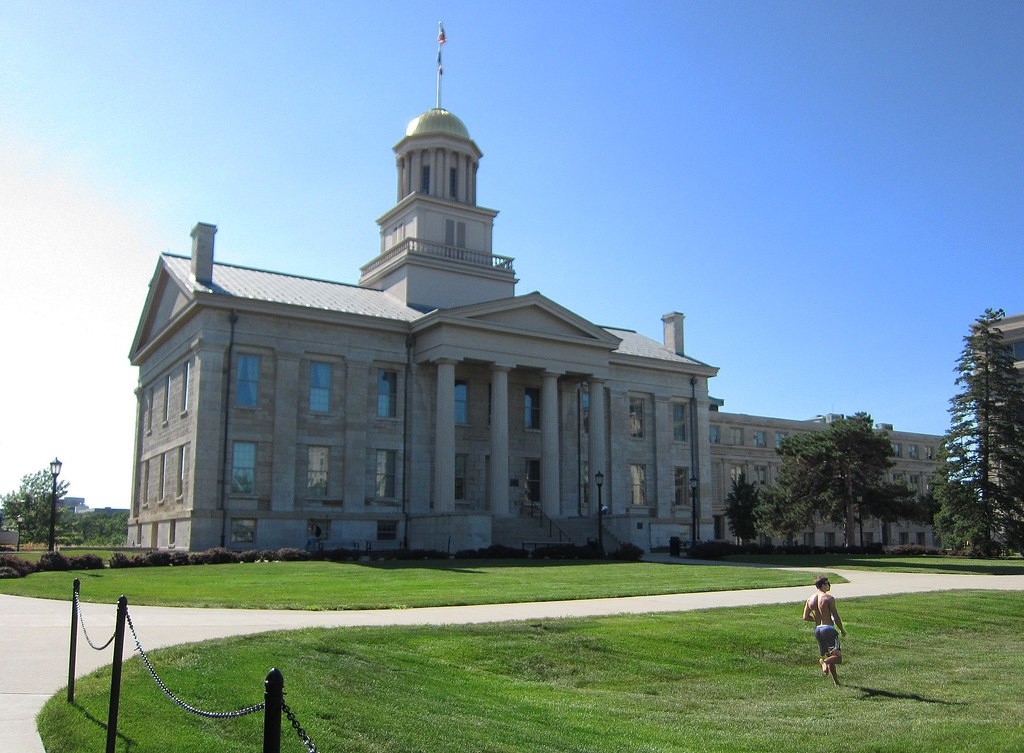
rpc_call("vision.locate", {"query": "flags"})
[437,29,447,44]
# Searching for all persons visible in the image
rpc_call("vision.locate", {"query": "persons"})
[803,576,846,686]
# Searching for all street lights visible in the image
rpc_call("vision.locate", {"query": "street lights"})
[48,456,62,552]
[689,475,699,544]
[594,470,605,560]
